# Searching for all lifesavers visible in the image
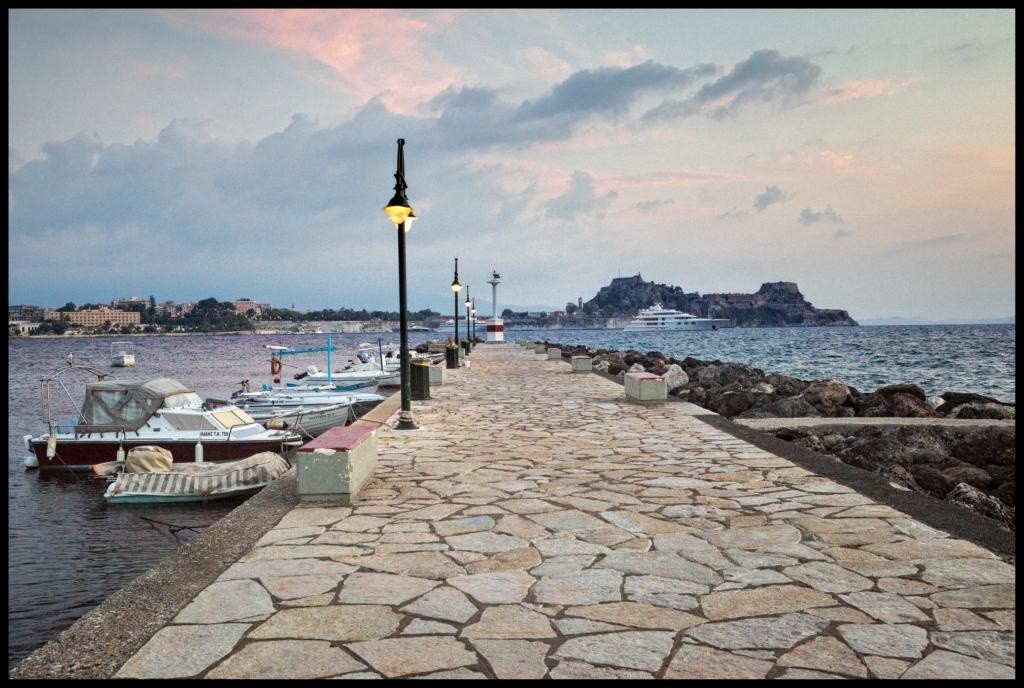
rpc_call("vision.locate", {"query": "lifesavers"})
[272,360,282,373]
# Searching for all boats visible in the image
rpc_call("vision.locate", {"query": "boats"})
[625,301,731,330]
[110,341,135,366]
[265,379,379,394]
[232,401,352,436]
[24,351,306,469]
[391,322,431,333]
[94,445,291,504]
[233,380,385,421]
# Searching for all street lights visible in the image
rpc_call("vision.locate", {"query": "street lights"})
[471,309,477,347]
[380,184,418,430]
[464,299,472,342]
[450,280,462,346]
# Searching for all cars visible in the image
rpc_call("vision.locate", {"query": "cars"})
[290,341,446,392]
